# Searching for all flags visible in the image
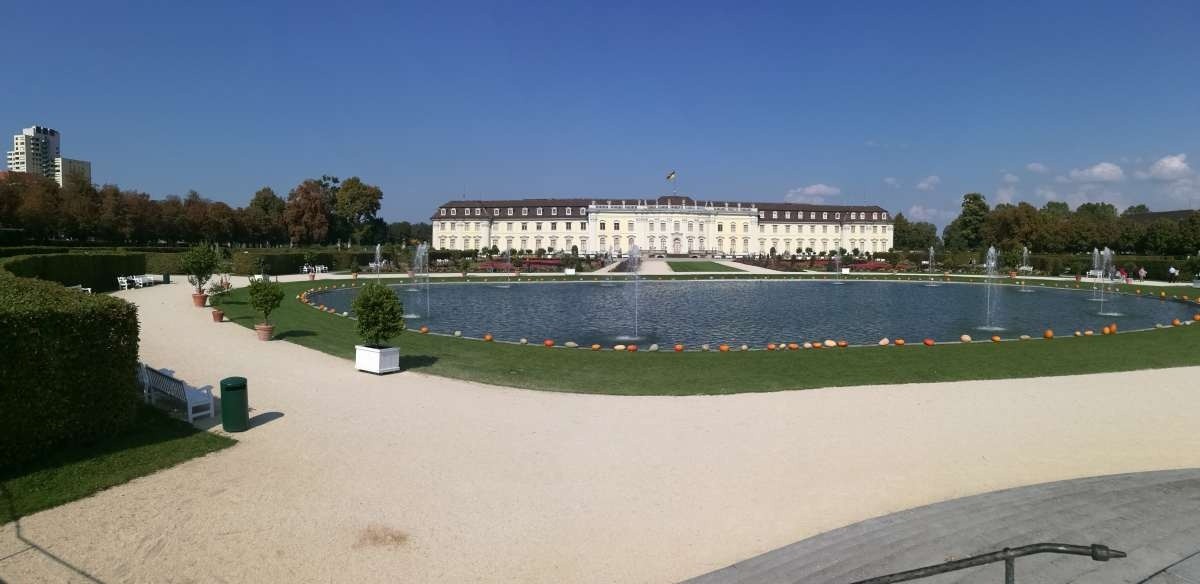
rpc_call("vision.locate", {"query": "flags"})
[666,170,677,181]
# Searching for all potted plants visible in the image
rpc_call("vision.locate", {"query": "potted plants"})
[1122,262,1138,284]
[248,257,272,283]
[179,241,219,307]
[1186,252,1200,288]
[461,260,469,277]
[512,257,523,275]
[941,259,953,276]
[351,282,404,377]
[218,262,233,291]
[560,255,578,276]
[206,282,228,322]
[350,264,359,279]
[1005,250,1021,278]
[1071,261,1084,282]
[249,278,285,342]
[841,254,852,275]
[403,254,415,279]
[306,252,316,282]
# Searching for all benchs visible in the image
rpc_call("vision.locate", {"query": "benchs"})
[1086,270,1107,279]
[1108,270,1126,280]
[1018,265,1034,275]
[136,359,152,396]
[116,275,142,291]
[130,275,154,288]
[298,264,315,275]
[65,285,92,295]
[143,362,215,424]
[314,263,330,273]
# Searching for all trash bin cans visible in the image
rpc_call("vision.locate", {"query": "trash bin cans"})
[219,376,249,432]
[163,271,170,284]
